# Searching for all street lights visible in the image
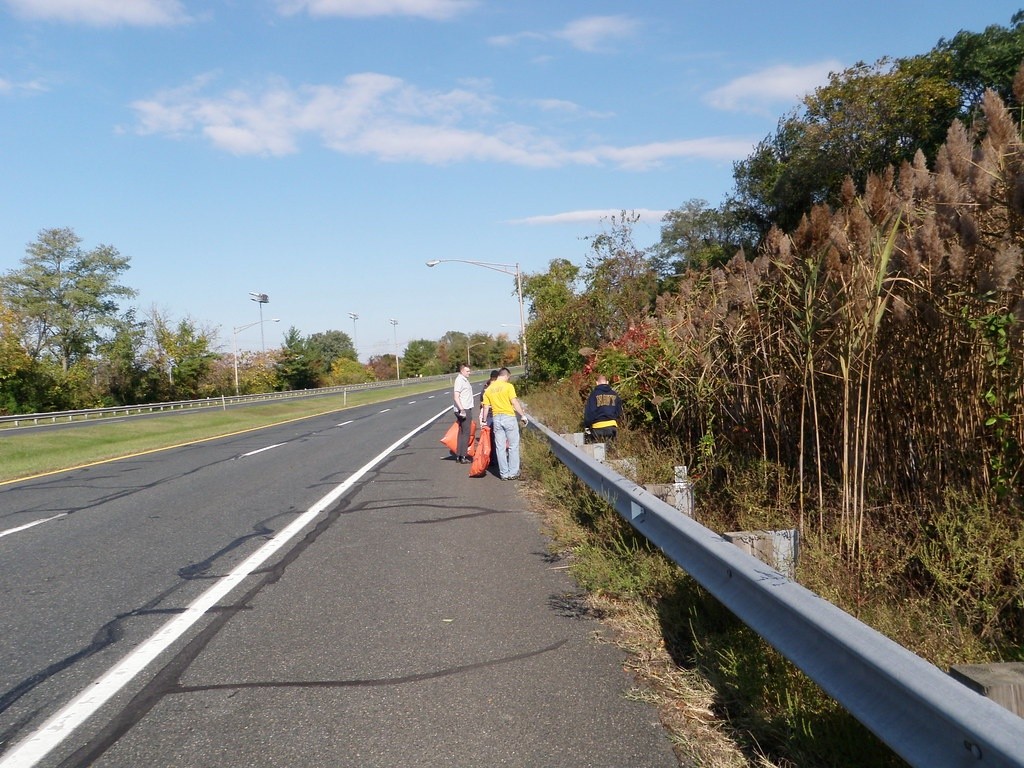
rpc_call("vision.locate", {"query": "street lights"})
[233,319,281,397]
[346,311,359,350]
[249,291,267,369]
[423,257,529,380]
[390,319,400,381]
[467,342,486,366]
[501,323,523,366]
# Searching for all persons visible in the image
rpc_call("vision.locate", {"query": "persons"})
[454,364,472,464]
[480,368,528,481]
[585,375,623,452]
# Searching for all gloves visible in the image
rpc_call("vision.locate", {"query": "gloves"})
[585,428,591,434]
[521,416,528,427]
[460,410,466,422]
[482,419,487,425]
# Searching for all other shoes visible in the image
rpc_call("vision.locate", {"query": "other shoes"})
[501,478,508,480]
[456,456,461,463]
[508,473,520,480]
[461,455,471,464]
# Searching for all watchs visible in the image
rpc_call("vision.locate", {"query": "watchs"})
[482,420,486,423]
